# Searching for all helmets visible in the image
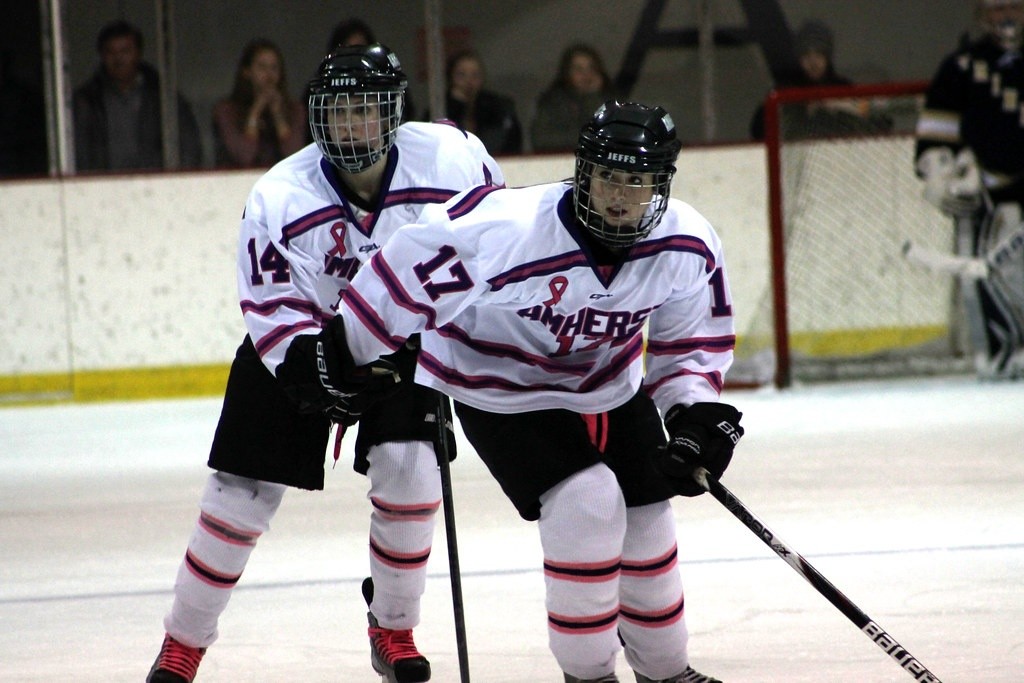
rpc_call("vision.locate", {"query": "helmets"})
[306,41,408,171]
[573,100,681,246]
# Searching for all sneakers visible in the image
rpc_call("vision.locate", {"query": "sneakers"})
[147,632,206,683]
[632,664,724,683]
[362,576,432,683]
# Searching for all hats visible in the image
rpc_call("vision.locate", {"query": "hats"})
[794,22,834,57]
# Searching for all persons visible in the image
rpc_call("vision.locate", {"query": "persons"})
[749,20,894,142]
[211,38,306,168]
[422,48,524,158]
[275,100,744,683]
[257,65,257,68]
[300,18,416,146]
[915,0,1024,379]
[147,42,506,683]
[68,20,203,172]
[529,43,614,155]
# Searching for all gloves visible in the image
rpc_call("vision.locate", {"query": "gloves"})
[654,404,743,497]
[275,314,371,405]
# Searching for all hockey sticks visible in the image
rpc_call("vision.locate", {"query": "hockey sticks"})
[425,393,475,683]
[898,223,1022,278]
[661,442,944,683]
